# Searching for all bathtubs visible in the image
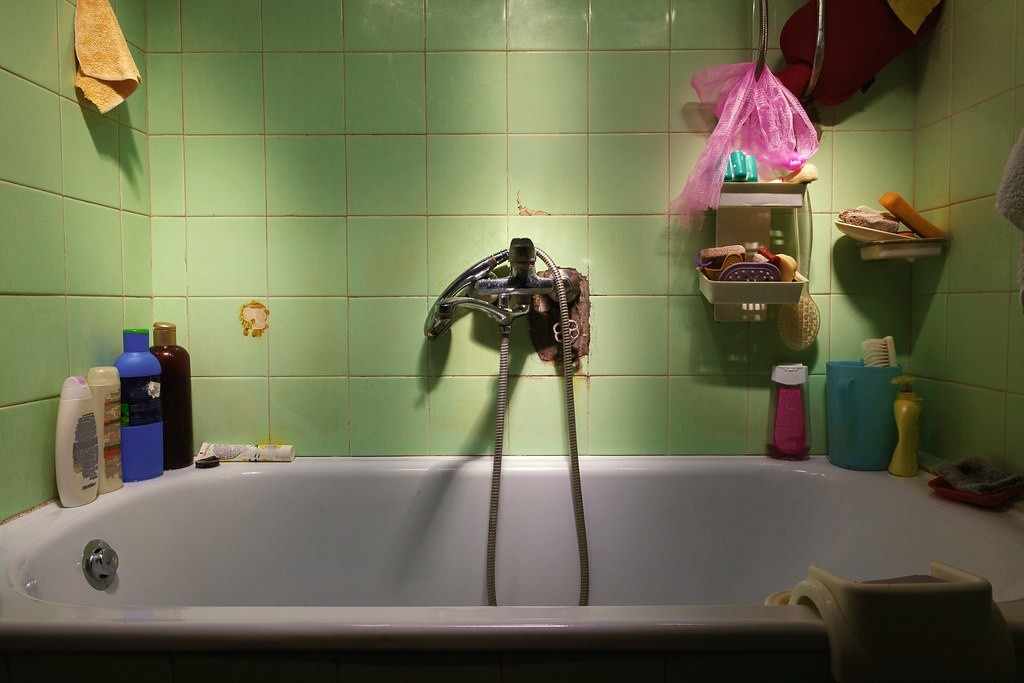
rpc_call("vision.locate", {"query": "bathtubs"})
[1,441,1024,683]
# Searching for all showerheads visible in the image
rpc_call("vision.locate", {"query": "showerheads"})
[423,256,498,342]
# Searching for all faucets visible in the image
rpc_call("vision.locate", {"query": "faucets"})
[432,296,535,332]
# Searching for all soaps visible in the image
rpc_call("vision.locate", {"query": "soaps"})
[195,451,218,470]
[715,150,760,183]
[770,255,799,284]
[880,192,945,239]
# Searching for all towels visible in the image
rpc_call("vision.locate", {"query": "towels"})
[73,1,143,117]
[994,128,1024,314]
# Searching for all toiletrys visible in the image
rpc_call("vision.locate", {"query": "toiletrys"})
[763,359,814,462]
[80,363,126,497]
[110,326,166,484]
[145,319,195,474]
[53,376,103,507]
[889,375,923,479]
[197,440,298,462]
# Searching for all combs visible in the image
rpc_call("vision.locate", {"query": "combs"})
[774,182,824,352]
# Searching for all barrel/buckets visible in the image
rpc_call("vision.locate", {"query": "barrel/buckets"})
[826,359,905,470]
